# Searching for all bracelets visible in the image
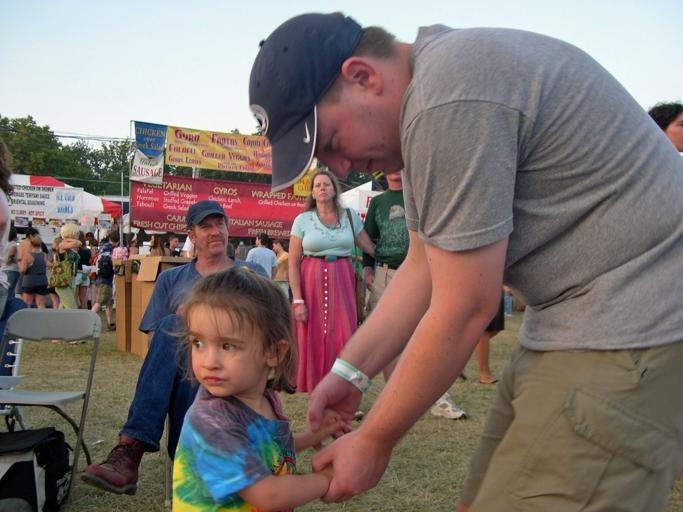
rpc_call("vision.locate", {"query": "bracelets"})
[335,359,373,394]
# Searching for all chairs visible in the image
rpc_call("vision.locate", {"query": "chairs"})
[0,264,101,511]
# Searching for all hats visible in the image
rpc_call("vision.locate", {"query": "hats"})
[184,199,224,230]
[247,11,363,196]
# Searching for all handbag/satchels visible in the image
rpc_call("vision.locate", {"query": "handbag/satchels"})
[354,271,367,322]
[46,250,73,289]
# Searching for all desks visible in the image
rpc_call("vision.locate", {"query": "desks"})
[113,256,199,362]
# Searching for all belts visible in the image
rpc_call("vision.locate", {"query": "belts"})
[377,261,400,271]
[304,255,350,262]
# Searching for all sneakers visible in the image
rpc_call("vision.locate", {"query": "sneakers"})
[428,391,467,420]
[51,323,116,345]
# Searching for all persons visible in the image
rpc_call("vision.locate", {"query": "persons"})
[646,102,683,152]
[459,290,507,385]
[246,10,683,512]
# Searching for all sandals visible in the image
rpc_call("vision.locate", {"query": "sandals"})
[459,373,499,384]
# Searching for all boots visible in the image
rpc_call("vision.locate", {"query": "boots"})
[78,433,145,496]
